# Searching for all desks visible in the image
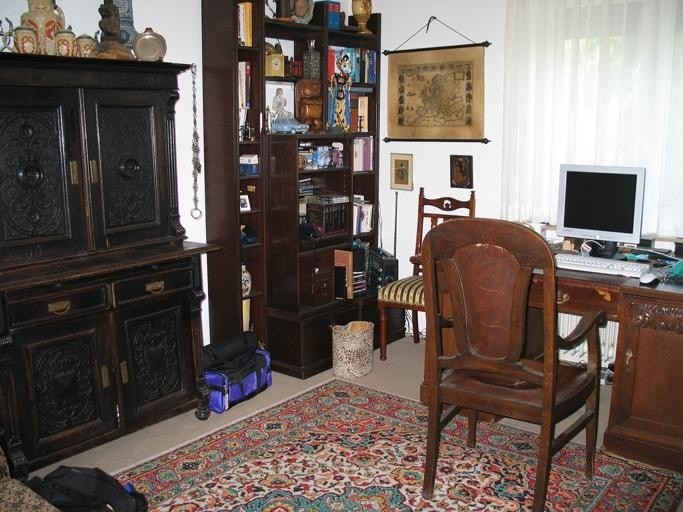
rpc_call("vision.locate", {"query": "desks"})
[419,240,683,473]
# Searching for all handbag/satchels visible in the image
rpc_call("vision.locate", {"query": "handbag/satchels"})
[203,331,272,413]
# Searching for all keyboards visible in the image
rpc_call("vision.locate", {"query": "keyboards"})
[554,253,651,278]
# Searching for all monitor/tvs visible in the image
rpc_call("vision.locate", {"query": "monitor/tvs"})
[556,163,647,261]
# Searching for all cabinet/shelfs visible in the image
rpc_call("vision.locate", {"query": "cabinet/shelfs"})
[0,242,222,483]
[0,51,191,280]
[262,130,405,380]
[201,0,380,345]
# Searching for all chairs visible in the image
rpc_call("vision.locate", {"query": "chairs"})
[420,218,607,512]
[377,187,476,360]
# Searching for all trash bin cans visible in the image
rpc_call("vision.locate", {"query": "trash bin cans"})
[331,320,375,380]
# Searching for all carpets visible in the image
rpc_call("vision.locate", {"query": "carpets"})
[110,376,683,512]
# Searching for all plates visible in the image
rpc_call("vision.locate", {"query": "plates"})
[131,32,166,60]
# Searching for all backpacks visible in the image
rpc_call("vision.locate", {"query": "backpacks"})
[26,465,148,512]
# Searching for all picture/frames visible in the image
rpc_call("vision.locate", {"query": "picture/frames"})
[450,155,473,189]
[240,195,251,211]
[390,153,414,190]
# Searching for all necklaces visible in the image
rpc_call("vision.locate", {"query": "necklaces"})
[189,65,201,221]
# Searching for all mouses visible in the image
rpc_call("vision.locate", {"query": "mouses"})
[640,273,657,286]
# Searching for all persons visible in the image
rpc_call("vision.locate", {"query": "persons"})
[335,53,351,75]
[395,163,407,181]
[271,88,292,118]
[451,158,469,187]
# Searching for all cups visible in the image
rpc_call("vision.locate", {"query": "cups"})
[53,31,77,56]
[74,34,98,57]
[2,25,38,54]
